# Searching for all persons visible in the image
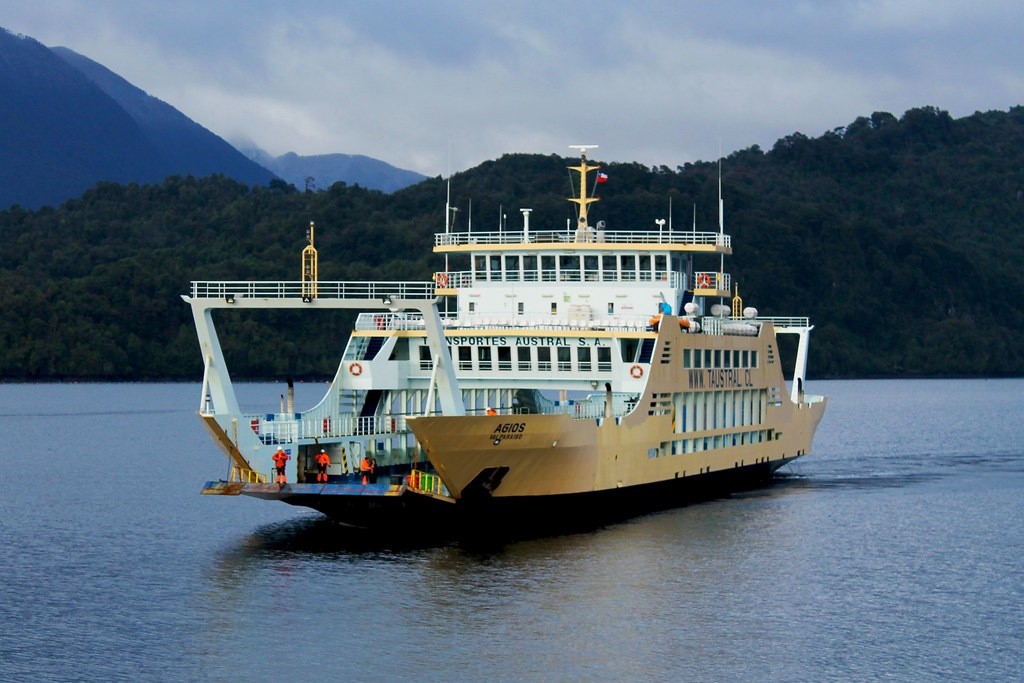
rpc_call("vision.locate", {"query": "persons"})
[486,407,496,416]
[315,449,330,482]
[361,457,377,486]
[272,446,288,483]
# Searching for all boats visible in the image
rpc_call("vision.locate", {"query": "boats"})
[180,144,826,538]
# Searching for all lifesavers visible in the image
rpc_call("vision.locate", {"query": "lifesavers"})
[438,273,449,287]
[697,274,711,288]
[629,365,643,379]
[349,362,362,377]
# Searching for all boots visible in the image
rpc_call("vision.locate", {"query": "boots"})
[363,476,367,485]
[317,474,321,481]
[323,474,327,481]
[282,475,286,482]
[276,475,280,483]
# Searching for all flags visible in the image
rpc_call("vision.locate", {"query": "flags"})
[597,173,608,182]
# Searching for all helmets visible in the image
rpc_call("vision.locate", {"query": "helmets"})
[486,407,491,412]
[320,449,325,453]
[277,446,283,451]
